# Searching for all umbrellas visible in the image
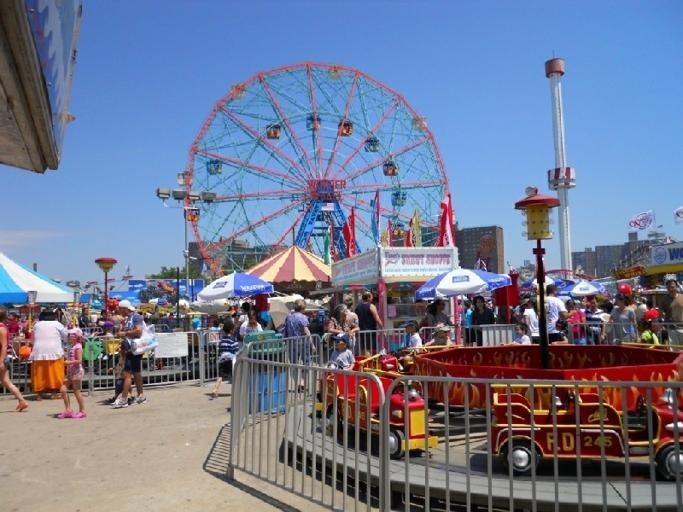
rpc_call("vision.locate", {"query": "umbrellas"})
[194,270,279,307]
[412,265,512,345]
[0,251,78,310]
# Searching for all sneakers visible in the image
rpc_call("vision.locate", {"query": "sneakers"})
[55,410,73,419]
[97,395,146,409]
[15,401,28,412]
[72,411,87,418]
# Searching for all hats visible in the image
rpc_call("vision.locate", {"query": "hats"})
[400,321,419,329]
[67,327,83,338]
[118,299,135,311]
[331,331,350,343]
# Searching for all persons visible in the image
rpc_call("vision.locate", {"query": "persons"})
[1,288,386,421]
[389,279,682,350]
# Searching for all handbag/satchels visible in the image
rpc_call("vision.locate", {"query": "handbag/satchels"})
[219,359,232,377]
[129,316,159,356]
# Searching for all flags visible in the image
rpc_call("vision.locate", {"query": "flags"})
[326,189,457,263]
[625,210,656,234]
[673,206,682,224]
